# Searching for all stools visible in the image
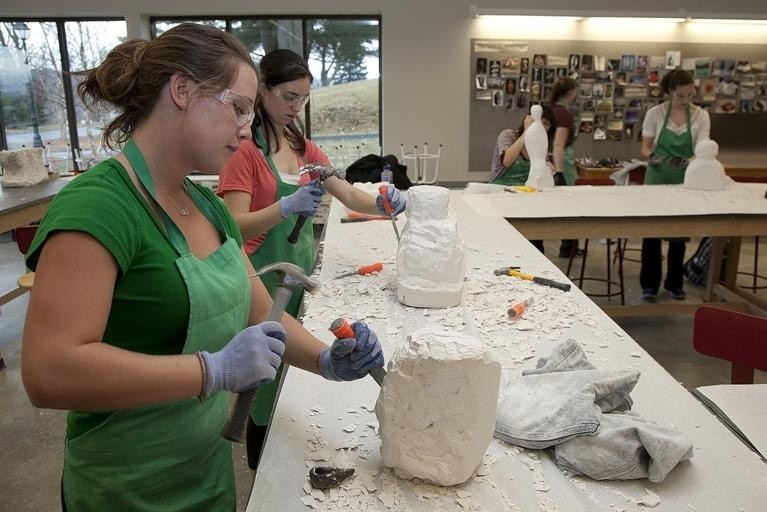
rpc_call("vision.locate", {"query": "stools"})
[613,165,665,270]
[732,177,767,294]
[339,142,366,169]
[20,141,52,175]
[398,139,445,185]
[65,141,87,172]
[565,179,630,306]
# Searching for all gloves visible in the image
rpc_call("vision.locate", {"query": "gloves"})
[318,321,384,383]
[554,172,565,186]
[199,321,288,405]
[378,184,405,219]
[649,151,660,169]
[667,154,689,172]
[281,177,325,220]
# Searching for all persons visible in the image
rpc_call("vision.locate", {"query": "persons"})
[217,49,406,485]
[545,77,589,257]
[639,69,711,301]
[22,23,384,512]
[489,106,557,256]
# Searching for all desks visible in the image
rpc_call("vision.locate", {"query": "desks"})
[0,173,80,308]
[244,183,767,512]
[575,162,624,179]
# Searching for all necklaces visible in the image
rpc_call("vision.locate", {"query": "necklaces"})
[151,173,191,216]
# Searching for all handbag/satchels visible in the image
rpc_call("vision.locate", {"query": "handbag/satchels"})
[687,237,730,288]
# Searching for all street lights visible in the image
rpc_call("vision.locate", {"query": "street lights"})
[13,20,44,148]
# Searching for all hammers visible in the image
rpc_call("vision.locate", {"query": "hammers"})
[288,161,346,245]
[219,262,320,444]
[494,266,571,293]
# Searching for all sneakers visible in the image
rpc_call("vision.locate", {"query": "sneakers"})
[642,285,660,299]
[560,247,586,261]
[663,285,686,301]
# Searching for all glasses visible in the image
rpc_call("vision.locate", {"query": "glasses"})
[197,86,256,128]
[670,92,695,103]
[266,82,311,109]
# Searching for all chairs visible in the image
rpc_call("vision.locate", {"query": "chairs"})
[693,303,767,384]
